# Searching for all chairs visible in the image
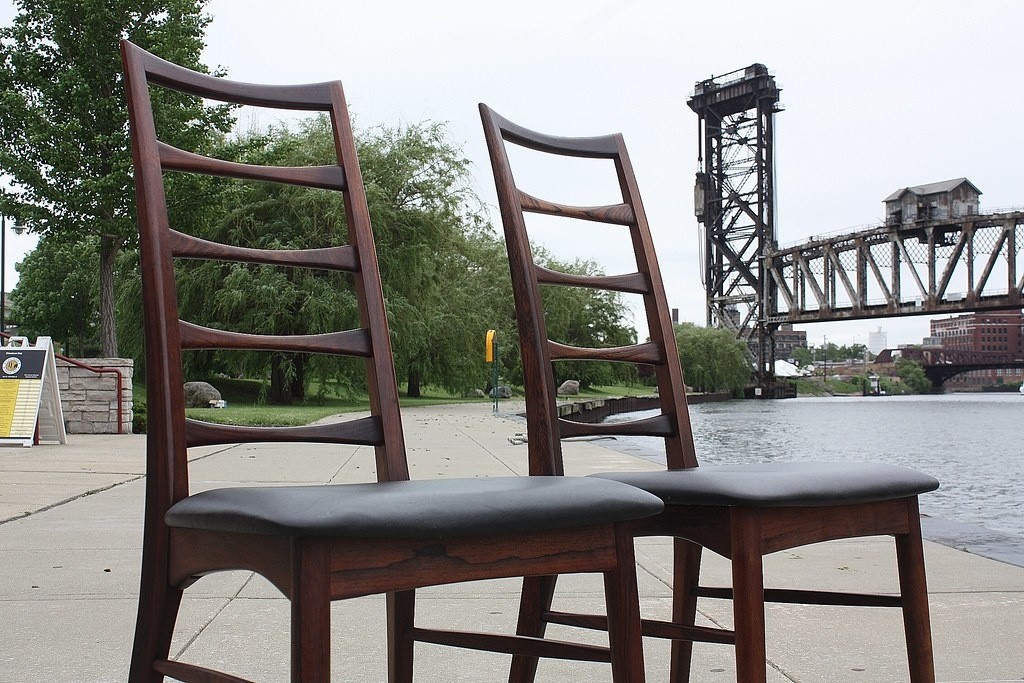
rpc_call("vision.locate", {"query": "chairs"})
[118,37,666,683]
[477,103,943,683]
